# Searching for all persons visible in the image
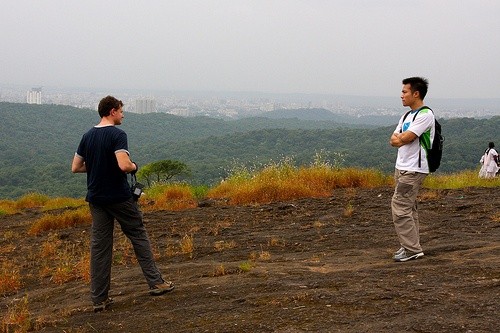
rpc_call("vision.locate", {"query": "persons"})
[479,141,500,178]
[72,95,174,312]
[390,77,435,261]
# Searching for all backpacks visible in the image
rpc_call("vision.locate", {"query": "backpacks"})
[400,106,443,173]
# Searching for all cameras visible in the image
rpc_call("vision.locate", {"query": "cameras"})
[129,182,144,201]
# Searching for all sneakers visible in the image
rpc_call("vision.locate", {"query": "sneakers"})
[93,298,113,312]
[392,246,424,262]
[149,280,174,295]
